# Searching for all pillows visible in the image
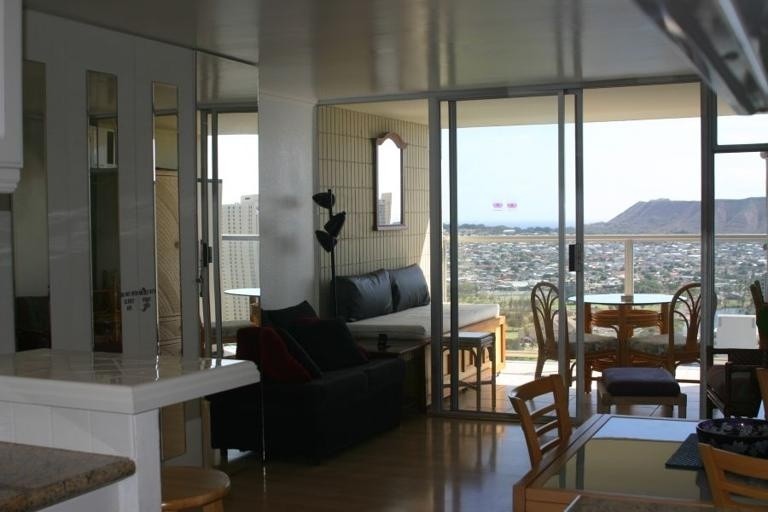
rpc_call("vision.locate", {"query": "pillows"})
[388,264,430,311]
[234,325,310,381]
[260,300,318,330]
[333,268,393,322]
[294,315,364,372]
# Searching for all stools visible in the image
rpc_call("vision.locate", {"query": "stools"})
[160,464,230,512]
[442,332,497,409]
[597,367,687,419]
[202,320,256,351]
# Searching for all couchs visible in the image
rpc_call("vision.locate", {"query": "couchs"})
[330,263,506,402]
[205,301,405,467]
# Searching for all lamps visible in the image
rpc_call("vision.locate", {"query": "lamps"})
[312,188,347,317]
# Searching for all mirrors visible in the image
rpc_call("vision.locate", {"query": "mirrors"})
[371,131,410,231]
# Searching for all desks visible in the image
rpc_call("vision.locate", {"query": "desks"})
[512,413,768,511]
[0,439,136,512]
[225,287,260,321]
[567,293,688,394]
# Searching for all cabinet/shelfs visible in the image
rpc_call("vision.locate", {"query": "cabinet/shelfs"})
[0,346,261,511]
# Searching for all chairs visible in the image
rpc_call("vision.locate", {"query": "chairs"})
[705,344,768,419]
[508,374,571,468]
[531,281,621,404]
[628,283,718,416]
[698,443,768,512]
